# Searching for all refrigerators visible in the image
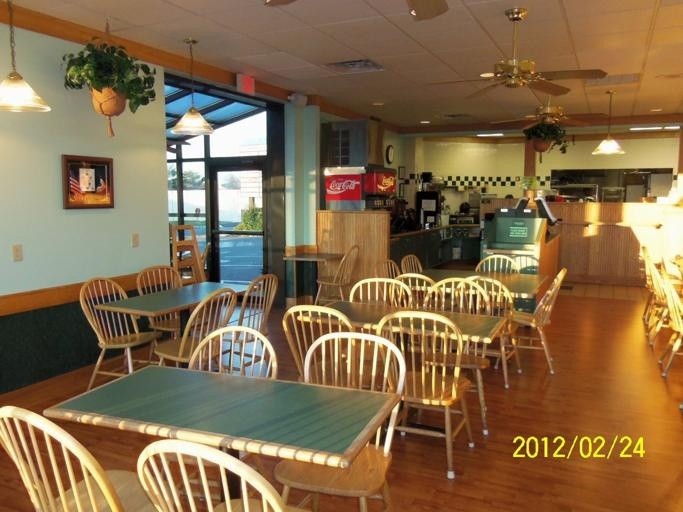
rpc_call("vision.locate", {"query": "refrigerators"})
[324,164,398,234]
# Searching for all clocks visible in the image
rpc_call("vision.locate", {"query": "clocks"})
[386,144,394,163]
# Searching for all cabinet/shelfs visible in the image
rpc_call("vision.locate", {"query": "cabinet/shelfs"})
[392,228,481,269]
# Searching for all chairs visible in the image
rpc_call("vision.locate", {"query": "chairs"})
[646,261,683,346]
[659,274,682,379]
[640,247,665,323]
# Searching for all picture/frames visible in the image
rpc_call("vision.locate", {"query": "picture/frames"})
[399,183,405,197]
[61,154,115,210]
[398,167,405,179]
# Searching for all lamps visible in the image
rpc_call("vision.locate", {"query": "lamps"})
[591,91,627,155]
[0,2,51,112]
[171,38,214,134]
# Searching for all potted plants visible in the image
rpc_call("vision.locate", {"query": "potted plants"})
[58,36,158,117]
[523,124,569,155]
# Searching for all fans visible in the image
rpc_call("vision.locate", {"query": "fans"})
[489,96,590,133]
[261,0,449,22]
[426,9,608,99]
